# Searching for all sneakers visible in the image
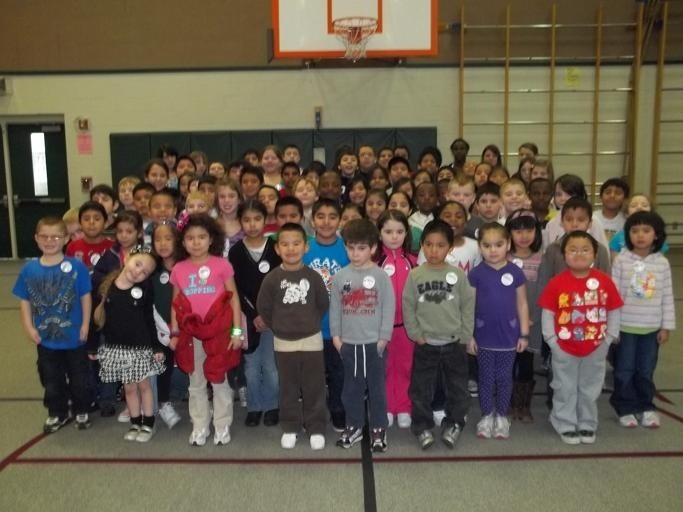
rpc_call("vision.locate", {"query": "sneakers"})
[213,424,232,445]
[561,431,581,444]
[159,402,181,429]
[188,427,211,446]
[580,429,596,443]
[332,411,468,450]
[492,414,509,438]
[617,414,638,427]
[636,411,660,428]
[476,414,492,437]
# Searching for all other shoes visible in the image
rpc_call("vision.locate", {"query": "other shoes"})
[262,409,280,426]
[119,407,131,422]
[245,411,262,426]
[468,381,478,397]
[280,432,297,448]
[135,424,157,441]
[123,424,140,440]
[45,416,67,432]
[75,413,91,431]
[100,406,116,417]
[239,386,247,408]
[310,434,324,450]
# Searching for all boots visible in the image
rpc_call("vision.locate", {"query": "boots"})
[523,380,536,422]
[511,382,524,422]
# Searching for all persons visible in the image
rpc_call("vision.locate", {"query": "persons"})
[11,134,676,453]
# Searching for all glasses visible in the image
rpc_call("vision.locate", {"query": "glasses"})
[36,233,66,241]
[564,246,594,255]
